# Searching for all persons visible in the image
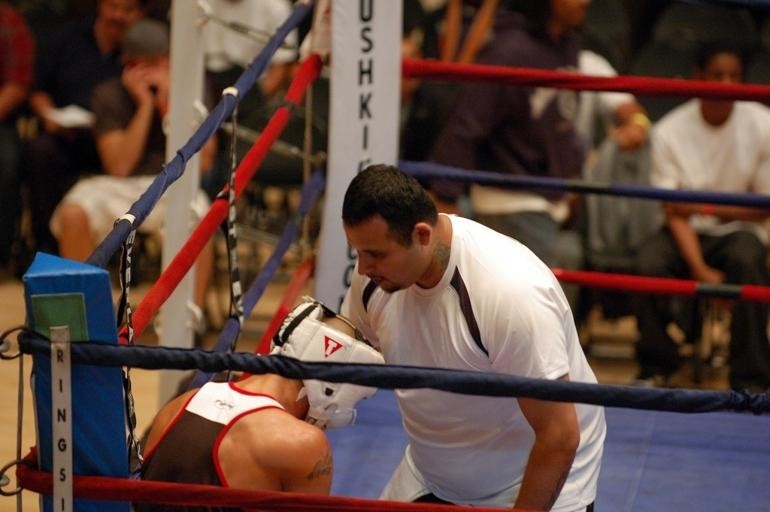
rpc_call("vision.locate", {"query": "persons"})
[132,297,387,510]
[338,163,607,512]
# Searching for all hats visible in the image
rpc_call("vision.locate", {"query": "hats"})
[119,17,169,59]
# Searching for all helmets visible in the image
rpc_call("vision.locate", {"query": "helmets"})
[267,298,387,431]
